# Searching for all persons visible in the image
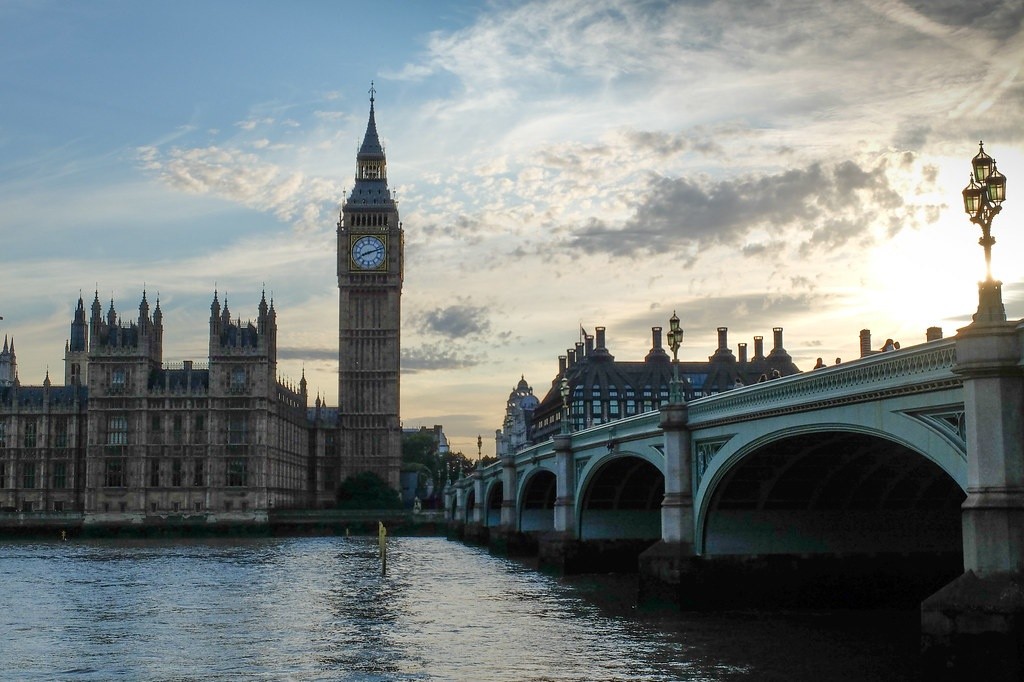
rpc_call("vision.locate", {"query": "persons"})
[413,498,420,515]
[549,435,553,440]
[881,339,900,354]
[733,378,744,389]
[758,370,781,383]
[814,358,841,370]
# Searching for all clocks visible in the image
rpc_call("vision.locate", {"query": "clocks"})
[353,237,385,269]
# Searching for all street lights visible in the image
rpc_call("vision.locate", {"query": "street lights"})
[961,140,1009,321]
[665,310,685,404]
[560,373,571,435]
[476,434,482,462]
